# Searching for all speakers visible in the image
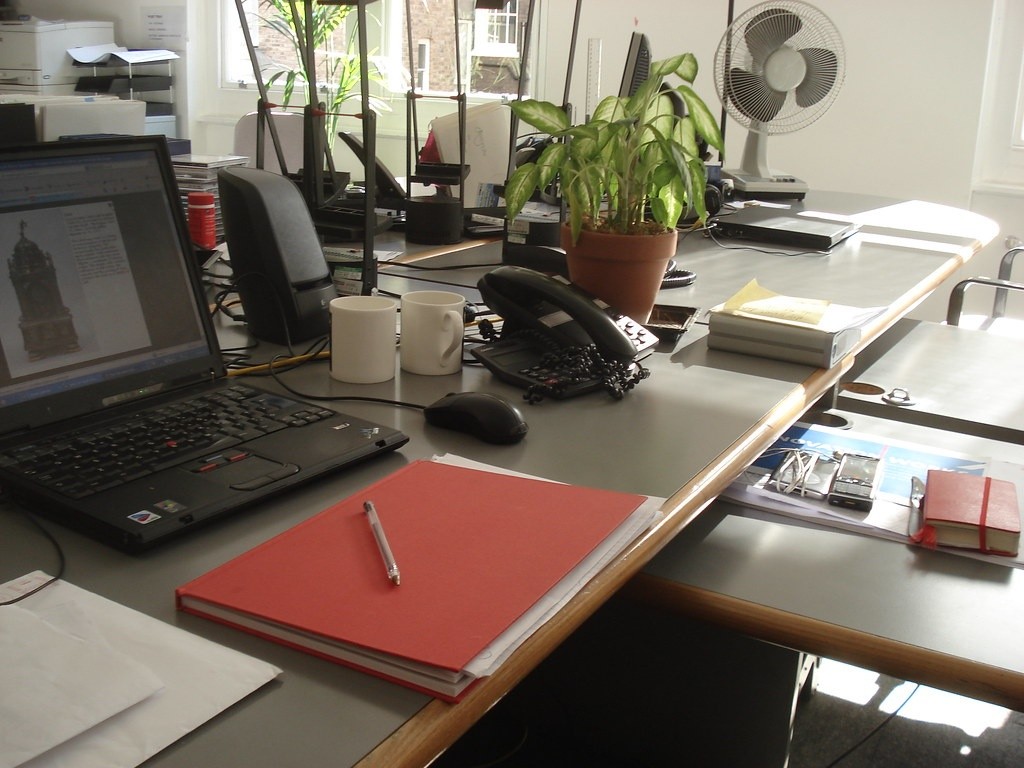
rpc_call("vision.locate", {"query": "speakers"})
[217,164,339,344]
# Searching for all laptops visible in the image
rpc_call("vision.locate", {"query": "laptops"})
[713,206,864,249]
[0,134,412,556]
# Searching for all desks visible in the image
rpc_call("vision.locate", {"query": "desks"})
[0,199,1024,768]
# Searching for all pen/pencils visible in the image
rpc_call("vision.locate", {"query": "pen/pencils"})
[362,500,401,587]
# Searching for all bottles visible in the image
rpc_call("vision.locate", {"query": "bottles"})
[188,192,216,251]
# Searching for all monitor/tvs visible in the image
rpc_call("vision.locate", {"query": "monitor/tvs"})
[616,32,686,134]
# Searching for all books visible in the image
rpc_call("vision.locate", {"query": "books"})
[921,470,1021,560]
[175,453,668,706]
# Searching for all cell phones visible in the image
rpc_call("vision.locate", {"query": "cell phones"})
[827,453,882,512]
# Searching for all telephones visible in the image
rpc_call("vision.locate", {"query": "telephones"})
[471,265,661,402]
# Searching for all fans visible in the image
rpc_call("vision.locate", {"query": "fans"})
[712,0,847,201]
[618,32,685,129]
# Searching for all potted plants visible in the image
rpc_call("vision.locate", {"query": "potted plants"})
[501,54,725,327]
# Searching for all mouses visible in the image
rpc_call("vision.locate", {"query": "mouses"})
[424,392,530,447]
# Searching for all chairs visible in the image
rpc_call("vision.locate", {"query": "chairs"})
[939,248,1024,334]
[232,111,304,174]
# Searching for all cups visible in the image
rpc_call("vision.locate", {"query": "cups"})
[400,291,464,376]
[328,296,397,383]
